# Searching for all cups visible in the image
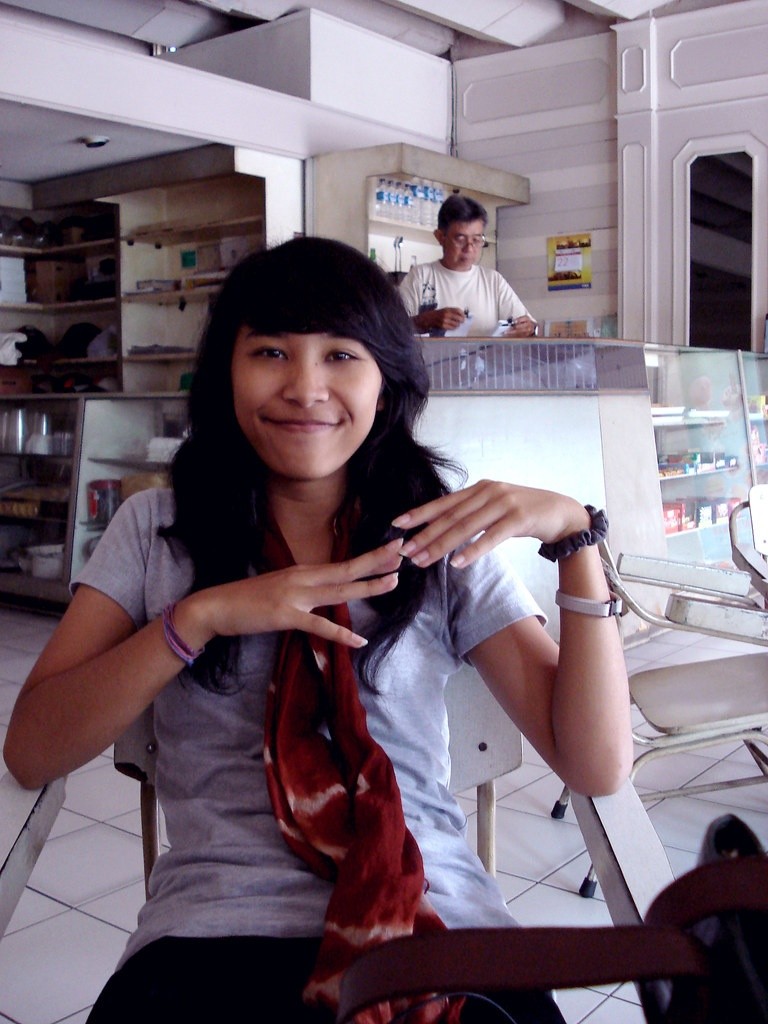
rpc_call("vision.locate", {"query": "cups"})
[0,408,73,456]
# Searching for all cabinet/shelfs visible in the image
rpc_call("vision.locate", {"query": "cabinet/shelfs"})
[0,142,303,621]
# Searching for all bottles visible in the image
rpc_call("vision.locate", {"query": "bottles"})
[375,178,445,229]
[370,248,377,265]
[410,255,416,270]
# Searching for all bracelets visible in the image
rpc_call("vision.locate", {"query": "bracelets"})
[555,590,623,617]
[538,504,609,563]
[161,601,205,664]
[529,324,538,337]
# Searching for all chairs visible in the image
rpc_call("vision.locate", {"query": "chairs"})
[0,484,768,1024]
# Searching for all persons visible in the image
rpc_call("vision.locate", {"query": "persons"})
[3,237,634,1024]
[397,195,541,337]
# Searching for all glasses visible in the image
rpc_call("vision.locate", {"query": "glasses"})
[441,231,486,248]
